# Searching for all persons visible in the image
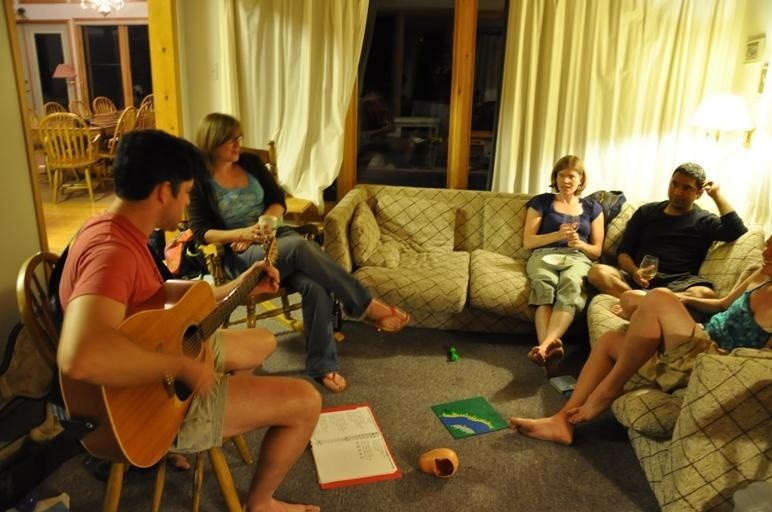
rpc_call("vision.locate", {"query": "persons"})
[609,236,772,353]
[187,114,410,392]
[509,287,772,445]
[56,130,323,512]
[587,163,747,299]
[523,155,605,377]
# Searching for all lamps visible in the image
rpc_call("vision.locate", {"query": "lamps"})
[52,63,84,89]
[78,0,129,20]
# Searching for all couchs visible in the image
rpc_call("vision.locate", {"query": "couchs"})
[583,280,772,512]
[321,176,772,336]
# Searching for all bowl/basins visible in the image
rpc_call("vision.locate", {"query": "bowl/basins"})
[541,253,574,271]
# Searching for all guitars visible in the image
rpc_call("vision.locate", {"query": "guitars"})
[58,234,281,469]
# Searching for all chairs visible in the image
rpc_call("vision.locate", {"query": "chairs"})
[202,129,319,329]
[36,109,108,206]
[27,91,156,179]
[15,247,257,511]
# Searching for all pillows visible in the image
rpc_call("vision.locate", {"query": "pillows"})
[350,196,380,266]
[480,196,536,262]
[370,191,457,252]
[611,388,680,440]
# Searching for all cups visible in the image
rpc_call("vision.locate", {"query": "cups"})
[259,214,277,239]
[638,255,660,283]
[563,213,580,232]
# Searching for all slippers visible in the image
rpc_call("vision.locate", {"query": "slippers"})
[321,371,346,394]
[362,306,410,331]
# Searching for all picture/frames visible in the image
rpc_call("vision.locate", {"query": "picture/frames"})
[742,36,766,63]
